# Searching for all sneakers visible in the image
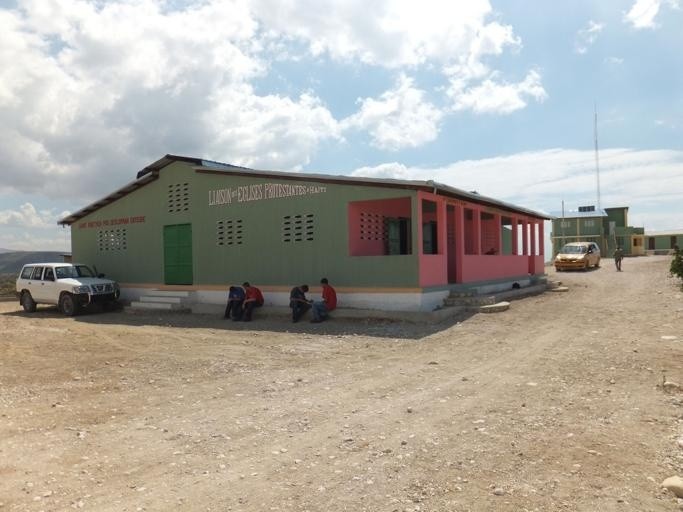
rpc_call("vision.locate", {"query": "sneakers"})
[310,319,320,323]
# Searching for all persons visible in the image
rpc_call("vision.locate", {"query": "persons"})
[309,278,336,323]
[288,284,314,323]
[483,248,497,255]
[612,246,624,272]
[231,282,264,322]
[221,286,245,322]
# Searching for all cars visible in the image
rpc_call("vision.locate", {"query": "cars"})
[554,242,600,271]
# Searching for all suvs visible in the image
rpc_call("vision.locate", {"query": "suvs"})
[16,262,120,316]
